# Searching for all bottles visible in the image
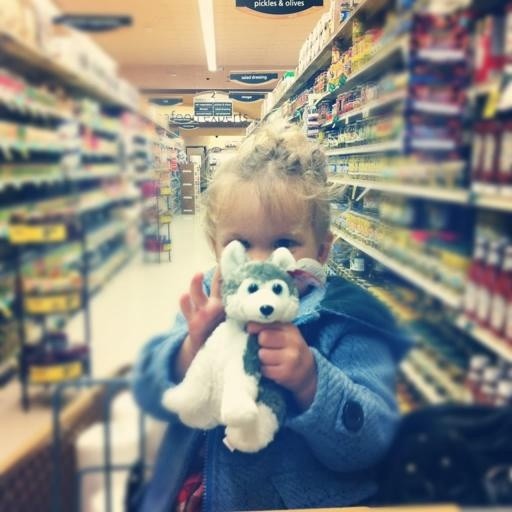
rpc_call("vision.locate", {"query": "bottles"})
[488,246,512,335]
[493,114,512,186]
[477,114,497,184]
[476,367,500,410]
[495,379,512,410]
[500,297,512,345]
[468,116,481,182]
[474,244,501,326]
[464,237,488,318]
[462,356,490,410]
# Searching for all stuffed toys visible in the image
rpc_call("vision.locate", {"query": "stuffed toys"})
[160,238,300,456]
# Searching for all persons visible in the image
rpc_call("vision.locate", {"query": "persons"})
[130,125,425,512]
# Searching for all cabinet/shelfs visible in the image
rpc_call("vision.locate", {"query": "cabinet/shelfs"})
[0,27,180,391]
[245,1,512,404]
